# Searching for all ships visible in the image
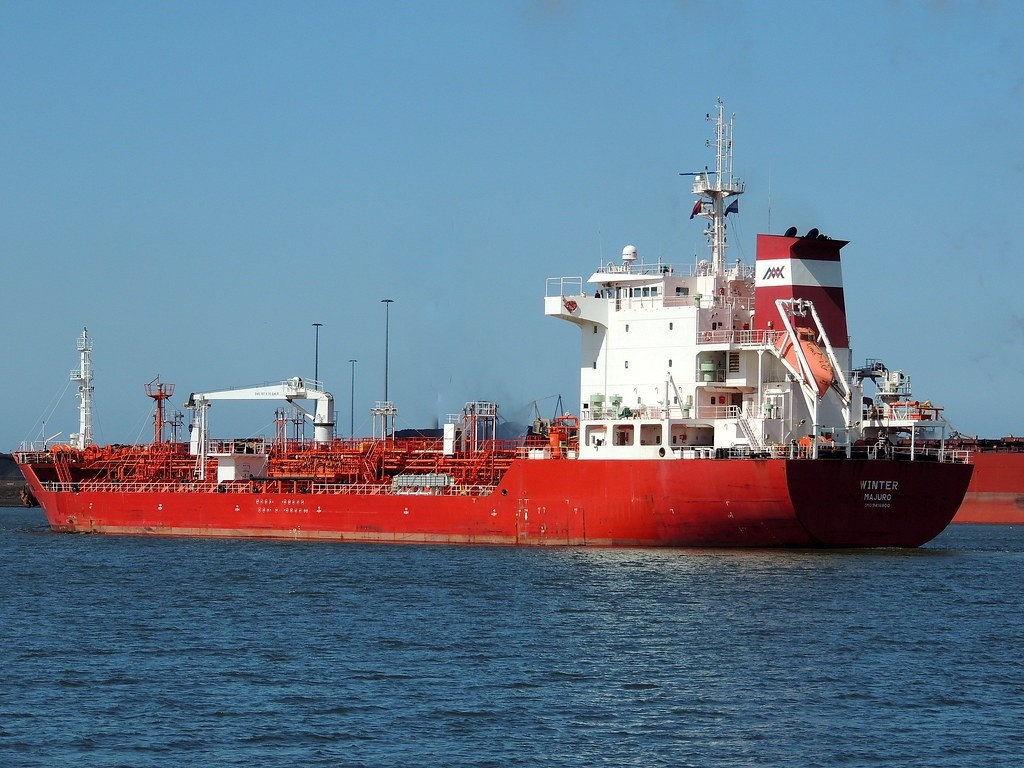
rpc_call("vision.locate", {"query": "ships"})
[10,95,976,549]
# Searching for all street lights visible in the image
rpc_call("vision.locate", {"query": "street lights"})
[348,360,358,441]
[380,299,394,439]
[312,323,323,442]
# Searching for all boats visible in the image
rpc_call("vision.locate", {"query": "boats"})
[775,330,835,398]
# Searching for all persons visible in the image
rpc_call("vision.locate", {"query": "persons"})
[218,485,226,492]
[252,486,259,493]
[595,290,600,298]
[287,486,293,493]
[300,487,307,494]
[274,486,279,493]
[597,439,607,446]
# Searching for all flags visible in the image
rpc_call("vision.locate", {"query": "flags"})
[725,199,738,217]
[690,199,701,219]
